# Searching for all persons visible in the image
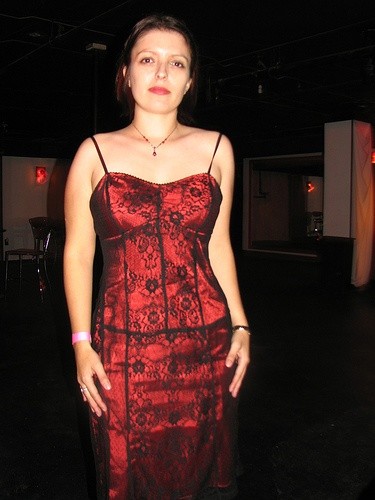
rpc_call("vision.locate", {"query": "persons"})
[62,15,252,500]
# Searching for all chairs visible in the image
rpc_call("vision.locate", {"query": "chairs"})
[5,217,57,288]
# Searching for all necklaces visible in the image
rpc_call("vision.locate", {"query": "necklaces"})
[131,121,178,157]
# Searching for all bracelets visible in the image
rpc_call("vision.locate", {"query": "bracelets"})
[231,325,251,335]
[72,331,92,344]
